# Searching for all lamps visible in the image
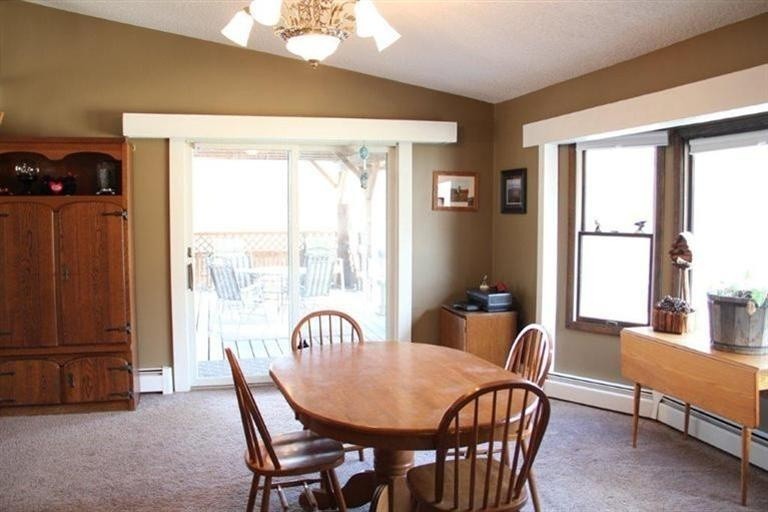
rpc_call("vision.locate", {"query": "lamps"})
[221,0,402,71]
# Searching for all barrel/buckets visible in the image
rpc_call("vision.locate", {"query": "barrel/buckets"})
[708,293,768,354]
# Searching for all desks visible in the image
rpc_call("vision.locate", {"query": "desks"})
[268,339,544,512]
[618,325,768,506]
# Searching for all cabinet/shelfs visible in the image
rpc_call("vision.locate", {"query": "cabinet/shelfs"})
[0,135,140,415]
[440,305,518,370]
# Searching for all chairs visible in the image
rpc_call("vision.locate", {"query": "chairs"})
[291,310,366,462]
[209,251,334,359]
[446,324,554,512]
[406,379,550,512]
[225,347,349,512]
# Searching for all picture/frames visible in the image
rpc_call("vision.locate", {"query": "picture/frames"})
[501,167,528,214]
[432,170,480,212]
